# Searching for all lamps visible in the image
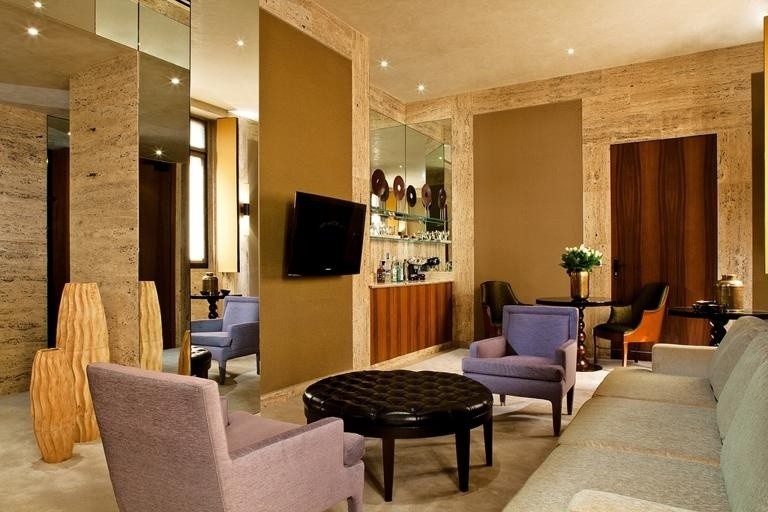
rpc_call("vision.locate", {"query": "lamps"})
[240,182,250,237]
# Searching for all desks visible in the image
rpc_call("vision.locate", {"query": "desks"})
[664,303,766,346]
[532,289,630,372]
[188,292,241,319]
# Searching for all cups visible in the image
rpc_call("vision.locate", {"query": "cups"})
[370,221,451,241]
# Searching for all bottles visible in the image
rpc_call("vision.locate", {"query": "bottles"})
[202,272,218,294]
[376,252,409,283]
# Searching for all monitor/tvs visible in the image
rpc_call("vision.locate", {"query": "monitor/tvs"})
[287,190,367,278]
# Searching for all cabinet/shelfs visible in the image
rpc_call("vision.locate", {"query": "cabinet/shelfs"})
[371,207,407,239]
[406,213,448,241]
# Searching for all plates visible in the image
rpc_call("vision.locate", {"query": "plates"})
[199,290,220,295]
[692,300,722,313]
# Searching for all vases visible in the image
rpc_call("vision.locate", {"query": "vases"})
[29,345,76,463]
[200,272,217,296]
[177,327,191,377]
[56,282,108,442]
[138,278,165,376]
[569,270,588,299]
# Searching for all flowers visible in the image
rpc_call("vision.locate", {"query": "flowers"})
[558,244,603,274]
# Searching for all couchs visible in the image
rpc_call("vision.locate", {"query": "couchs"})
[503,313,766,512]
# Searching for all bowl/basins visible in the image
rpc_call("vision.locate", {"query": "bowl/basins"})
[220,289,231,295]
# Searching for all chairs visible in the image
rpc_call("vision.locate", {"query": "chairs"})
[478,280,534,336]
[464,303,580,436]
[592,280,670,368]
[88,360,367,512]
[190,295,260,384]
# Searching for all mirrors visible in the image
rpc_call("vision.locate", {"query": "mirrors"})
[370,124,407,240]
[406,125,444,241]
[140,1,263,418]
[367,107,407,239]
[403,116,451,272]
